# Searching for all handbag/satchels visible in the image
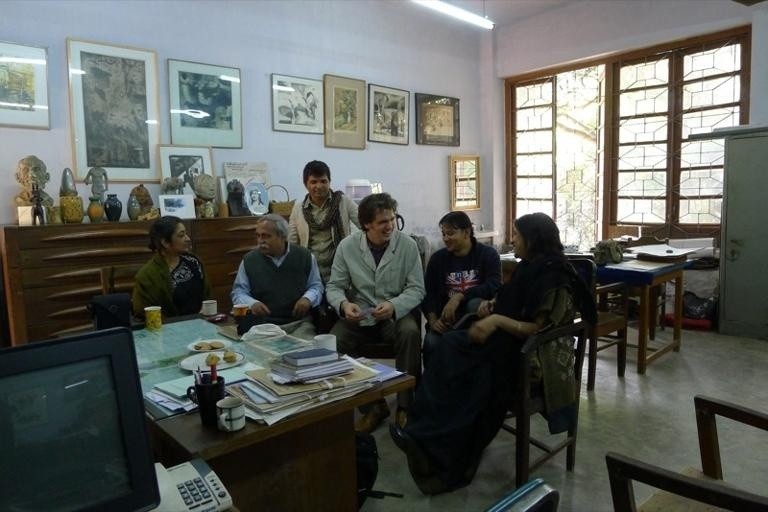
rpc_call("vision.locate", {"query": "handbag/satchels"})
[597,240,622,263]
[682,290,714,320]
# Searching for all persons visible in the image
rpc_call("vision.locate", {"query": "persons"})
[229,214,324,341]
[131,214,212,319]
[195,174,220,216]
[12,154,54,209]
[422,211,502,390]
[29,191,44,224]
[288,158,360,282]
[84,157,108,203]
[249,189,267,214]
[386,212,580,456]
[325,193,426,431]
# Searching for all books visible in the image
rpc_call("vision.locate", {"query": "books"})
[142,346,408,427]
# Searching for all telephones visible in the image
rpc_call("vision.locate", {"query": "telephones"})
[148,456,233,512]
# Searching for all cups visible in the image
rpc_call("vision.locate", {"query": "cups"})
[199,300,218,316]
[313,334,336,354]
[232,304,248,317]
[143,306,162,330]
[215,397,245,432]
[187,377,224,420]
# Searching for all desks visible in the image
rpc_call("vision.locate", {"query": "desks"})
[133,318,417,511]
[597,256,700,374]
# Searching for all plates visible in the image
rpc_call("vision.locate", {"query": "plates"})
[187,340,232,351]
[177,352,245,372]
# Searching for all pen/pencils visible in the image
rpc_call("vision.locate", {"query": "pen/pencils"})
[198,365,202,382]
[195,372,200,384]
[211,364,217,385]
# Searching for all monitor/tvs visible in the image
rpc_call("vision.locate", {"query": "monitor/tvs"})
[0,323,163,512]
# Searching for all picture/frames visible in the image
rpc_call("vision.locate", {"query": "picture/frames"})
[415,92,460,146]
[167,58,242,149]
[323,73,367,150]
[65,36,165,183]
[156,143,218,201]
[368,83,410,145]
[0,39,51,130]
[271,72,326,134]
[244,178,271,219]
[159,194,197,221]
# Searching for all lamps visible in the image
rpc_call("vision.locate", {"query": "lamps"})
[407,0,497,34]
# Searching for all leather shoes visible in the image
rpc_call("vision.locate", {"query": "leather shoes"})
[395,406,407,426]
[357,403,390,439]
[390,422,410,451]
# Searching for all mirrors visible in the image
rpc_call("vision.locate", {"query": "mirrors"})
[447,153,483,213]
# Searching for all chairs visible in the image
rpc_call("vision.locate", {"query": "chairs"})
[324,233,427,382]
[480,476,560,512]
[97,263,143,294]
[562,259,630,391]
[606,394,768,511]
[453,259,597,489]
[608,236,671,340]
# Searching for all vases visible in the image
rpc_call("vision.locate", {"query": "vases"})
[104,195,124,222]
[86,195,105,223]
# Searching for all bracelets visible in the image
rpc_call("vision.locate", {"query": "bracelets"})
[518,322,522,338]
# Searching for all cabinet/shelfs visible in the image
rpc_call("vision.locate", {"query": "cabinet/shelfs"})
[194,215,291,317]
[1,217,194,347]
